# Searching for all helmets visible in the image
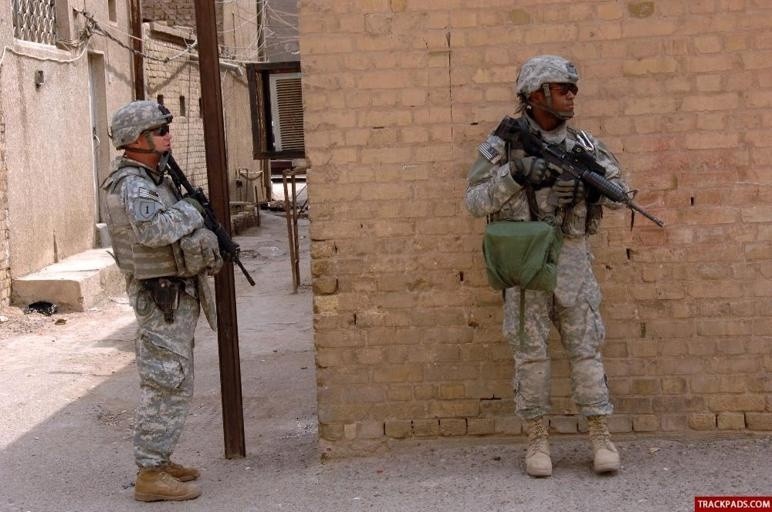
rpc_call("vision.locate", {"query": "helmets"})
[514,54,581,103]
[111,100,173,150]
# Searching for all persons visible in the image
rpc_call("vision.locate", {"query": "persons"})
[462,53,630,476]
[102,100,225,503]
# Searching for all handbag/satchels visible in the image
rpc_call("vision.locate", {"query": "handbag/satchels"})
[480,217,561,295]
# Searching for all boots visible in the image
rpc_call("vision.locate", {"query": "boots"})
[584,412,622,473]
[519,414,553,478]
[133,458,202,502]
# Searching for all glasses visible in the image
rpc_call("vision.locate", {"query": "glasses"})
[548,84,580,97]
[149,124,170,136]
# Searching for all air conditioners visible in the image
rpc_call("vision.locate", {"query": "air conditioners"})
[266,74,309,156]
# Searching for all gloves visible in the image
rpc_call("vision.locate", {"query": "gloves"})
[510,154,563,185]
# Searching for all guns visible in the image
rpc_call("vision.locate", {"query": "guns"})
[162,150,256,286]
[144,280,178,321]
[492,115,664,228]
